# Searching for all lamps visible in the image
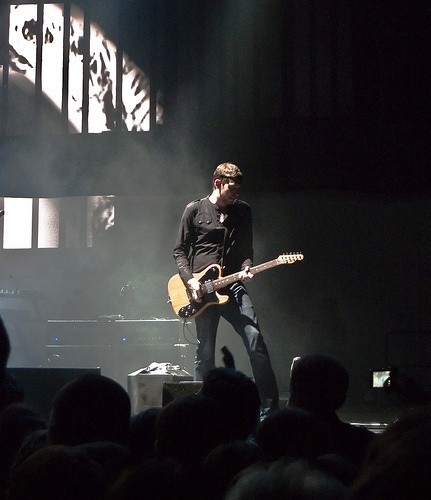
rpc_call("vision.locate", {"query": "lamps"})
[367,365,425,407]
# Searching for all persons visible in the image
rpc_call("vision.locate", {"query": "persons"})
[173,164,279,421]
[0,321,431,500]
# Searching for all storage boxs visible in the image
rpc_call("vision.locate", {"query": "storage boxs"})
[128,361,194,415]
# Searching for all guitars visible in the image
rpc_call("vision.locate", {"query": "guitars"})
[167,252,304,320]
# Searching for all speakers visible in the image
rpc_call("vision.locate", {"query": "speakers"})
[4,368,98,419]
[161,382,204,406]
